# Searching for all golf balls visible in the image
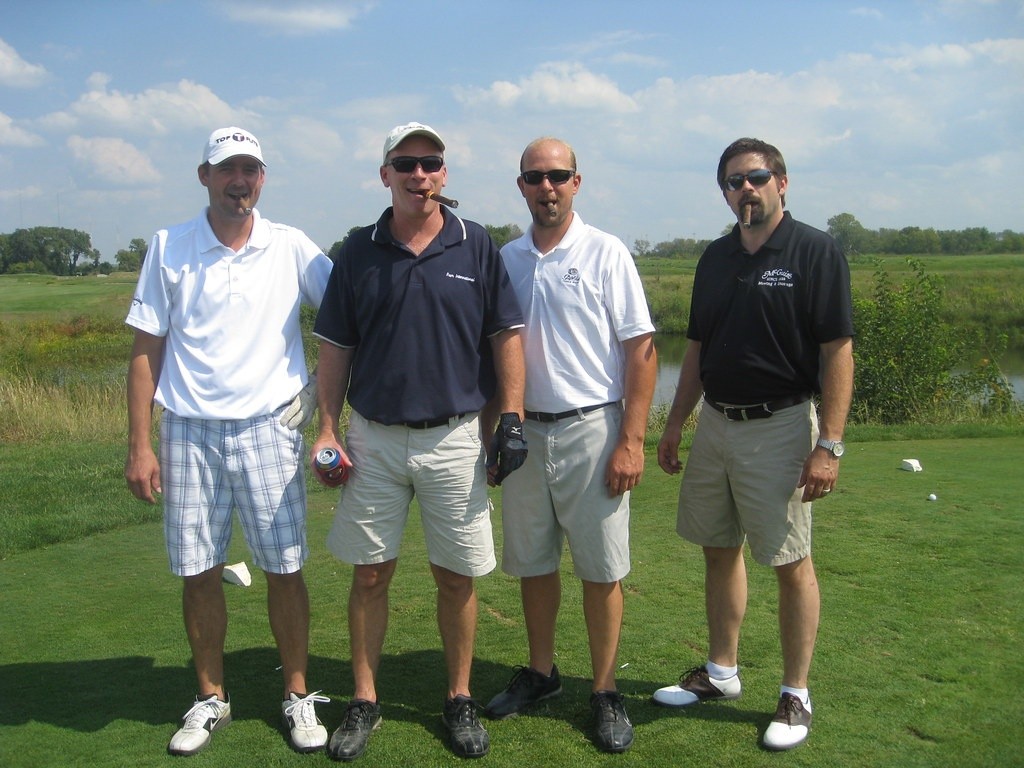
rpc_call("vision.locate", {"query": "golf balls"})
[929,494,937,501]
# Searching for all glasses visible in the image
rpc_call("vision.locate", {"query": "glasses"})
[723,169,778,192]
[385,155,443,173]
[521,169,576,186]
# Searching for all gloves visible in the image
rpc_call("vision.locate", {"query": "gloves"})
[280,373,317,432]
[484,412,528,486]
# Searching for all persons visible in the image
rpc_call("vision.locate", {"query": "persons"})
[485,136,657,752]
[652,137,855,751]
[124,126,352,754]
[311,119,528,760]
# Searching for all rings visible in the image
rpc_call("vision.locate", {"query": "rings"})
[823,488,830,493]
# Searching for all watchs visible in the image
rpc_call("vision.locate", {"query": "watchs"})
[816,439,845,458]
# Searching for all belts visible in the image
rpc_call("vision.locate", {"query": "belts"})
[704,392,812,421]
[396,414,464,429]
[525,402,615,423]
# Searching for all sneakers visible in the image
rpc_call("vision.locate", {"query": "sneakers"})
[280,688,328,752]
[327,698,382,760]
[590,690,632,752]
[485,664,563,720]
[169,692,232,755]
[440,693,489,757]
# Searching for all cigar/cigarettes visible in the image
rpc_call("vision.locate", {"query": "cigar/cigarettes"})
[420,189,459,209]
[235,195,252,216]
[546,202,557,218]
[743,204,751,229]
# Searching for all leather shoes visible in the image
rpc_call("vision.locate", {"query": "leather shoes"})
[652,665,741,704]
[762,690,811,749]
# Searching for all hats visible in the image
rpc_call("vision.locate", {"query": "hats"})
[383,121,445,161]
[201,127,267,168]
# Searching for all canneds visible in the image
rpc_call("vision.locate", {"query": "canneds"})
[315,448,349,488]
